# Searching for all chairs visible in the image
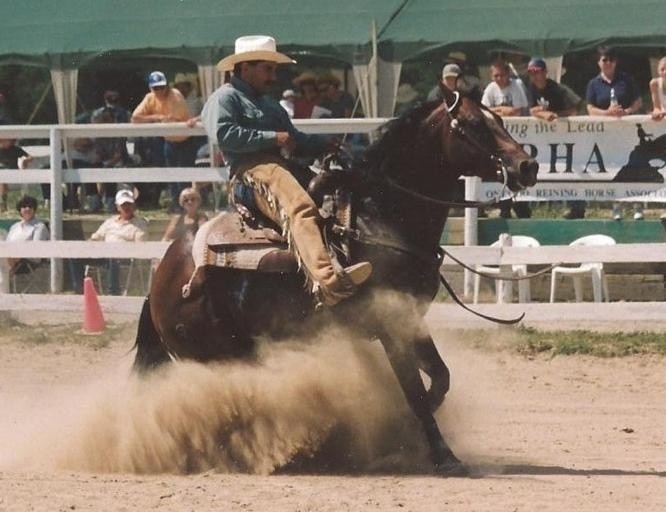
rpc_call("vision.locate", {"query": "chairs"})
[7,218,161,296]
[473,235,543,303]
[550,235,619,303]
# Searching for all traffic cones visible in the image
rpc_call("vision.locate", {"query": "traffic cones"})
[74,273,110,338]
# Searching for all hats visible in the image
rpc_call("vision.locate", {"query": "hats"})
[442,64,461,79]
[115,189,135,206]
[292,72,341,85]
[446,51,466,64]
[396,83,417,102]
[217,35,298,72]
[527,58,545,72]
[149,69,168,87]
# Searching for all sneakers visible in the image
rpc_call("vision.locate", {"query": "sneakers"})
[318,260,373,307]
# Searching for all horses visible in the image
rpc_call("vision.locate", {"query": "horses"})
[126,79,541,478]
